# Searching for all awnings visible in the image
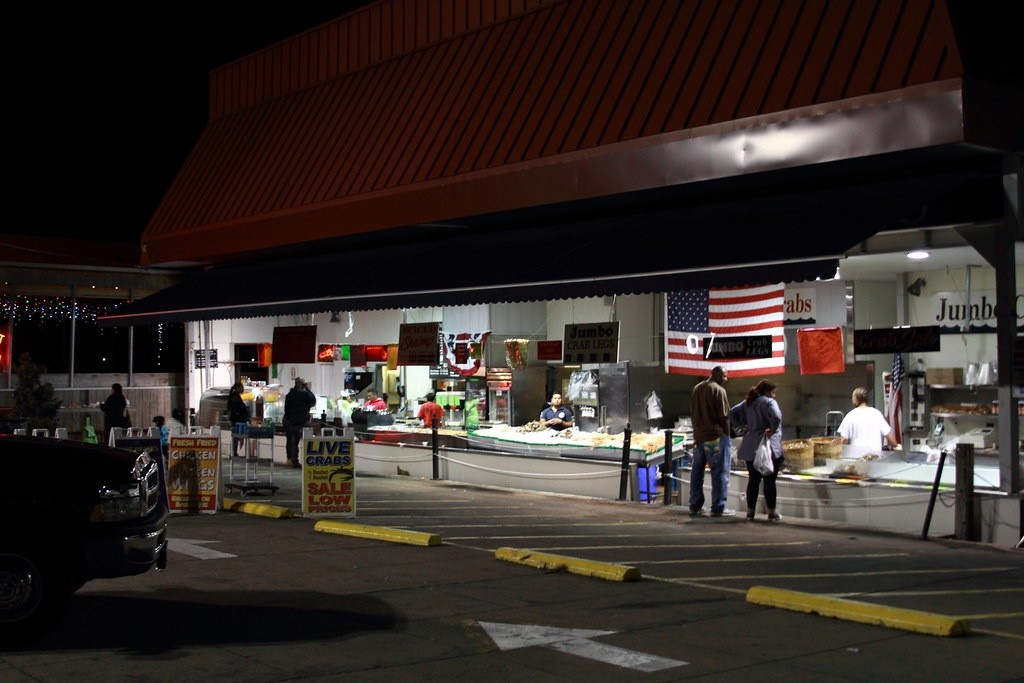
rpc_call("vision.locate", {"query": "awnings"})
[98,174,971,327]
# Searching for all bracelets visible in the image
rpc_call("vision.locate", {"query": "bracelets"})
[560,420,563,424]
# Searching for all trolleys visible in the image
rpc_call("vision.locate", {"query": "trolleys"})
[225,422,279,497]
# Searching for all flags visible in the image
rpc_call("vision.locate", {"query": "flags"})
[883,354,906,449]
[663,282,787,377]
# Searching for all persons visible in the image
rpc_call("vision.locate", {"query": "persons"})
[688,365,735,516]
[417,393,445,428]
[338,390,358,416]
[727,380,782,521]
[836,387,898,461]
[282,377,316,468]
[227,382,250,457]
[99,383,126,447]
[540,392,573,431]
[153,416,169,464]
[364,389,386,410]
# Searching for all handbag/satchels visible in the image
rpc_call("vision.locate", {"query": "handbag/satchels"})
[753,435,774,476]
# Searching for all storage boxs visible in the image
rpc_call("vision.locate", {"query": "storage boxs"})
[926,368,963,384]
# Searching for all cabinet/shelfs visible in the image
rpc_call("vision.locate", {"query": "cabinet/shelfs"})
[230,424,279,497]
[929,384,1024,456]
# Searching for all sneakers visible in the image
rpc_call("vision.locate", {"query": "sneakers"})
[747,513,755,521]
[690,504,708,514]
[711,508,736,516]
[767,513,782,522]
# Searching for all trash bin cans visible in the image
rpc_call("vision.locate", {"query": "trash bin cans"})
[637,463,657,500]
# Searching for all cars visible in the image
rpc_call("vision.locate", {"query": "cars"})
[0,434,169,623]
